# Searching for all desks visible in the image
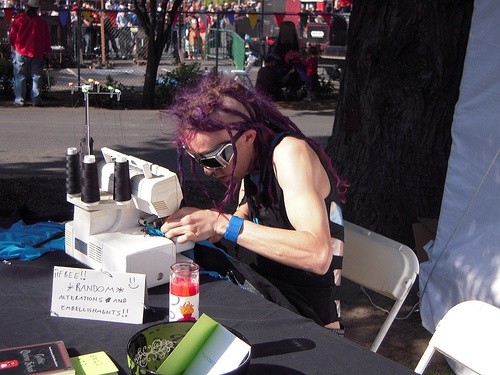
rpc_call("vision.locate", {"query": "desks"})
[0,210,424,375]
[318,55,346,90]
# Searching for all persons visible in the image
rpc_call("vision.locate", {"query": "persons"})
[0,0,352,109]
[161,69,349,337]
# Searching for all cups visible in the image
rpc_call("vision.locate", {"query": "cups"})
[169,262,199,322]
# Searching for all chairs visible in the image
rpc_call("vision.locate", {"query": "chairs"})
[343,219,419,353]
[414,301,500,375]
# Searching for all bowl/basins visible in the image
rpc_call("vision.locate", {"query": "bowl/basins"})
[126,320,253,375]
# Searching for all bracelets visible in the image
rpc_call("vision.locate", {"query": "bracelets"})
[224,216,243,242]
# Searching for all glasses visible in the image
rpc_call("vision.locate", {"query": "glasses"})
[182,128,244,170]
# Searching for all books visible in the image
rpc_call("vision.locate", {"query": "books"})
[0,340,119,375]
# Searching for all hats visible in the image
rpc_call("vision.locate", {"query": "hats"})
[27,0,40,7]
